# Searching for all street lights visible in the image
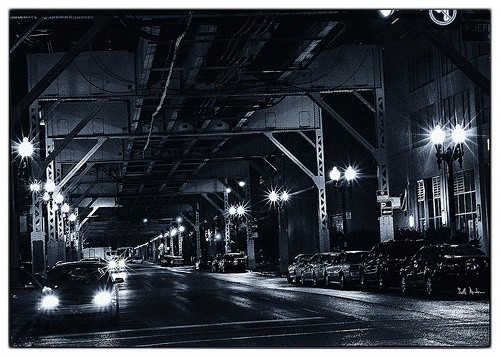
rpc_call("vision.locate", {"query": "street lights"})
[229,206,246,251]
[329,165,357,248]
[20,137,77,272]
[269,192,290,265]
[429,124,466,245]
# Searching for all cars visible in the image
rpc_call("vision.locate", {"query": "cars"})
[210,253,247,273]
[33,259,123,329]
[399,244,491,297]
[161,254,185,266]
[299,252,339,288]
[322,250,372,290]
[110,255,144,274]
[286,254,314,286]
[193,255,214,271]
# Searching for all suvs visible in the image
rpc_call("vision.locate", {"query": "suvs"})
[358,239,423,293]
